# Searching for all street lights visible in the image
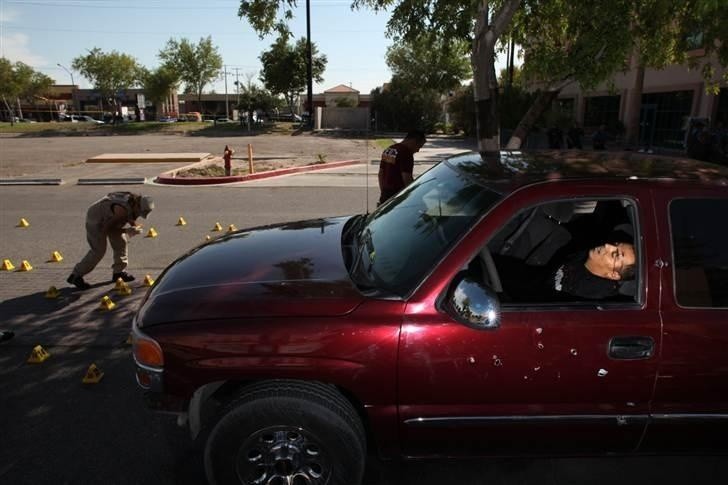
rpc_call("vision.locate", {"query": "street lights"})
[56,60,73,85]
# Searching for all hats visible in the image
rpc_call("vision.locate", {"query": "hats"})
[139,192,153,219]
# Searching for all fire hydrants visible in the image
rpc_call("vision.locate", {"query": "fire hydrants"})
[222,142,234,176]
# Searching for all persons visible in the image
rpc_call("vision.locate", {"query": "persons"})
[684,119,727,162]
[567,121,584,150]
[67,192,155,289]
[379,129,427,204]
[545,123,564,150]
[592,124,609,149]
[523,242,636,305]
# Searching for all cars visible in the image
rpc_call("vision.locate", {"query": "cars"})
[7,111,306,125]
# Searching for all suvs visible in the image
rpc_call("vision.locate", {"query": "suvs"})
[125,145,726,485]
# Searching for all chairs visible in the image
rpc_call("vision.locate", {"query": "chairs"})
[483,198,584,266]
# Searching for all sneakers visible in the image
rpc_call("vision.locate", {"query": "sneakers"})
[66,272,90,290]
[110,271,136,282]
